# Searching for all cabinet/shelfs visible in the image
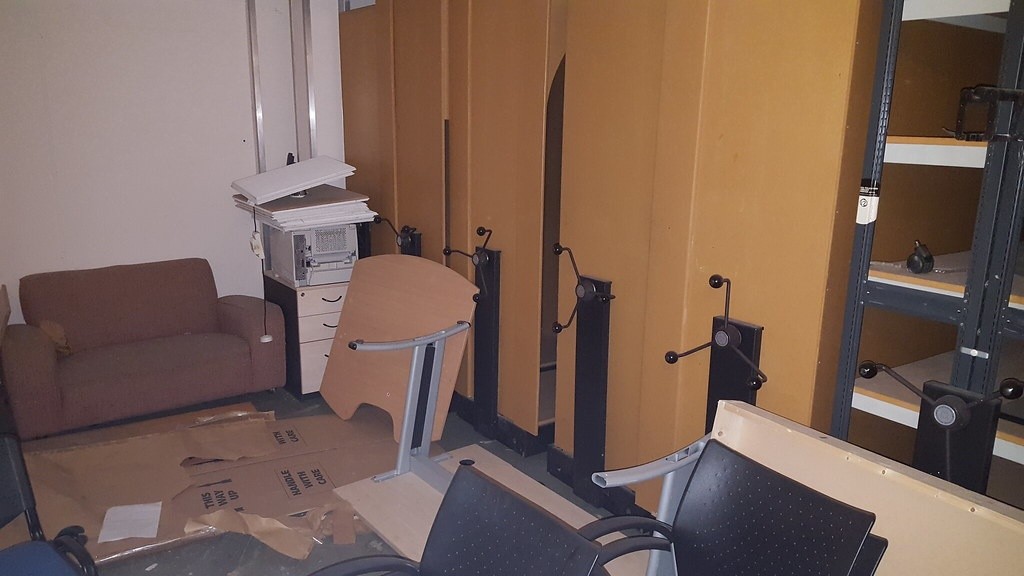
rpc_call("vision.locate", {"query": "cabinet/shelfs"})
[830,0,1024,496]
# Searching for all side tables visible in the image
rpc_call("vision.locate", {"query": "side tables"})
[263,270,349,401]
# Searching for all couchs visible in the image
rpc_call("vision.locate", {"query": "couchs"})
[0,258,287,443]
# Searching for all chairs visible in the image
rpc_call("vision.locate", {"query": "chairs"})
[0,359,98,576]
[307,439,889,576]
